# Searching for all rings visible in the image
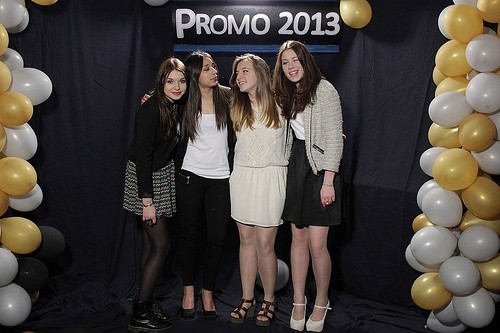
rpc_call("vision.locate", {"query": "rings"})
[325,203,328,205]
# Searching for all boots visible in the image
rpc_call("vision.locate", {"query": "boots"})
[128,298,174,332]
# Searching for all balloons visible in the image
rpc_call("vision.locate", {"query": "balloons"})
[406,0,500,333]
[0,1,58,326]
[340,0,371,28]
[145,0,168,7]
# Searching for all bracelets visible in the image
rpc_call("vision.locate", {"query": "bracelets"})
[323,184,334,186]
[143,203,152,207]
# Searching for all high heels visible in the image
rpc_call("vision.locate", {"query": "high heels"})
[230,297,276,326]
[181,291,217,321]
[290,295,332,332]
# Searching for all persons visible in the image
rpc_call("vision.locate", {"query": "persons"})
[122,59,190,332]
[228,53,293,326]
[271,40,344,332]
[140,50,232,316]
